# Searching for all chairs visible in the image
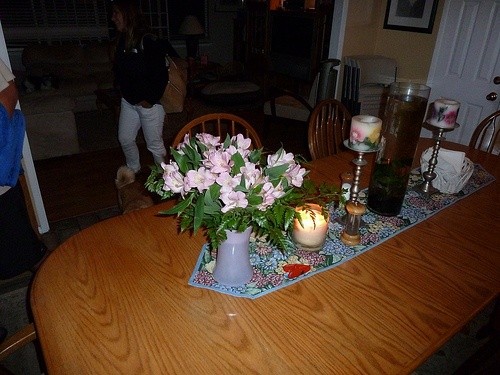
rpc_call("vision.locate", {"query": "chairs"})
[308,99,352,159]
[342,53,399,120]
[0,251,51,362]
[469,110,500,157]
[171,113,262,149]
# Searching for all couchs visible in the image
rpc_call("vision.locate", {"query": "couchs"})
[23,41,116,112]
[13,70,80,160]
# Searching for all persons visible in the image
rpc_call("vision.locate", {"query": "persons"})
[112,1,169,189]
[0,55,19,120]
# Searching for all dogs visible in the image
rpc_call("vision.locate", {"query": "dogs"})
[114,166,153,215]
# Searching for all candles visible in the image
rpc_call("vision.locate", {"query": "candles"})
[427,96,462,128]
[349,114,383,150]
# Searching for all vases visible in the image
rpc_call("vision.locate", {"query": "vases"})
[211,225,254,286]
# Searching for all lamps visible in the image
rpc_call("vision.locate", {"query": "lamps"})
[177,14,206,58]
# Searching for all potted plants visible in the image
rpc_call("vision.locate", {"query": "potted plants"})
[292,203,331,252]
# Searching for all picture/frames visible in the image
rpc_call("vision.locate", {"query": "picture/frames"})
[215,0,249,12]
[383,0,439,34]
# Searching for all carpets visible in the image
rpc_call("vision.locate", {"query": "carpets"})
[74,97,239,155]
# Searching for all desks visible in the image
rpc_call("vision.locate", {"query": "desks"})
[28,138,500,375]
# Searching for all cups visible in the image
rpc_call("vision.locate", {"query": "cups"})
[367,82,432,216]
[294,204,330,251]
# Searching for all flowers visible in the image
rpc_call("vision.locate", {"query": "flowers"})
[144,132,349,253]
